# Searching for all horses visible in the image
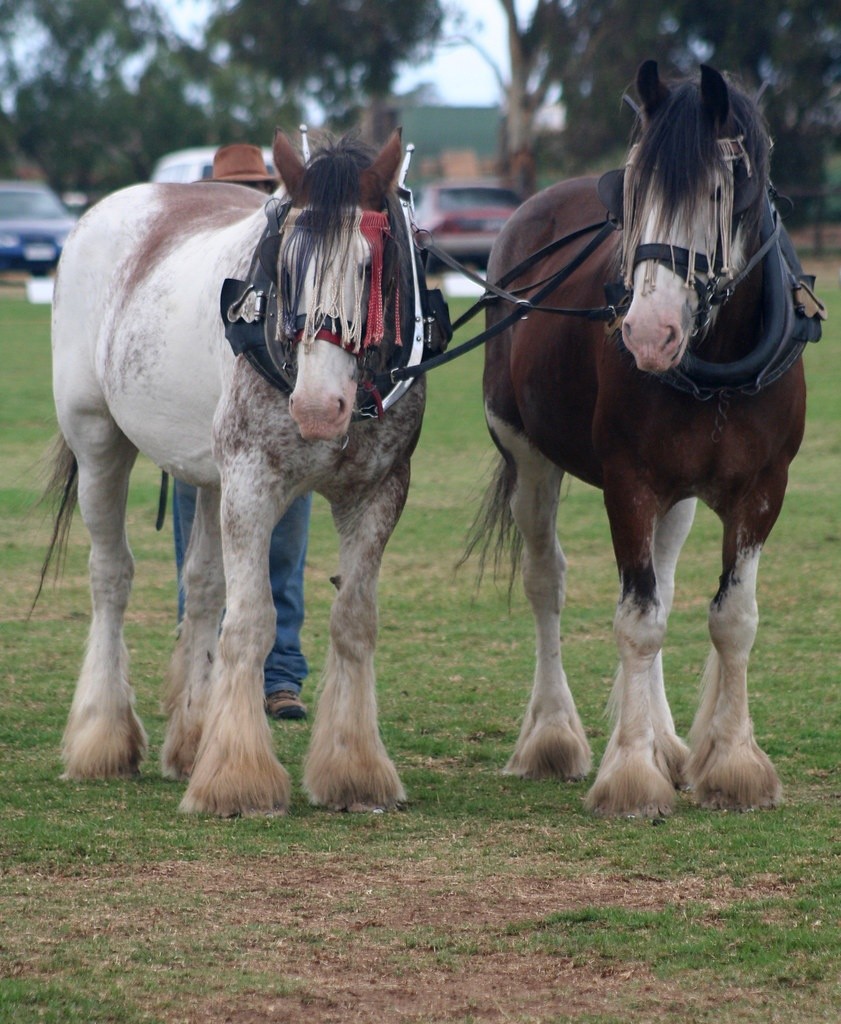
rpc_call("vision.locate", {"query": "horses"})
[22,122,428,824]
[448,57,807,822]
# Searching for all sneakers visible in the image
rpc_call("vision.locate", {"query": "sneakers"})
[266,690,307,719]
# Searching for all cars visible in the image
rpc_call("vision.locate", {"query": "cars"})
[1,178,76,280]
[414,183,528,273]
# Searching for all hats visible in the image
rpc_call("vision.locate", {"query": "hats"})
[194,143,280,185]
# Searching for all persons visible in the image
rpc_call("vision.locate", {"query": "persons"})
[172,147,316,720]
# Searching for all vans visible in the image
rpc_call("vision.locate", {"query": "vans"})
[151,146,280,185]
